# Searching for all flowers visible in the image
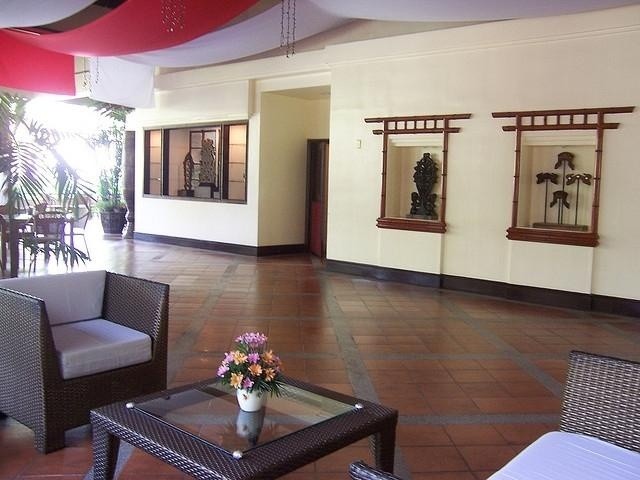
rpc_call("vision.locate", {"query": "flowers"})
[216,333,283,397]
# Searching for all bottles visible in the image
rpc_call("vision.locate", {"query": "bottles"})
[27,206,33,215]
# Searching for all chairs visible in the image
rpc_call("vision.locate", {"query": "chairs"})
[0,268,171,454]
[0,201,78,279]
[349,348,639,480]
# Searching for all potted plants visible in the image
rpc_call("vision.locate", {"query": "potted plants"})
[87,122,131,237]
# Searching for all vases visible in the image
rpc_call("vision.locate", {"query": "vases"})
[236,383,266,413]
